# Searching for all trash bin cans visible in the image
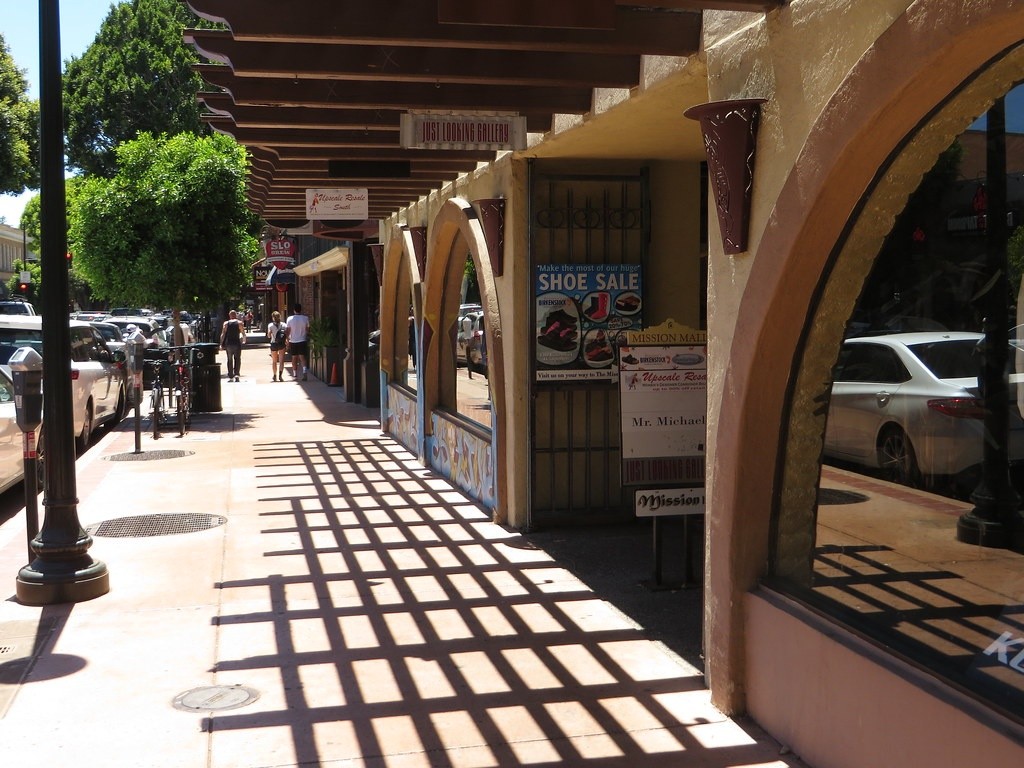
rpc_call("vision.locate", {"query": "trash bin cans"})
[185,342,223,413]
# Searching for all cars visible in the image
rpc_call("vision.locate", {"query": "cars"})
[456,303,488,377]
[824,331,1024,488]
[0,293,204,495]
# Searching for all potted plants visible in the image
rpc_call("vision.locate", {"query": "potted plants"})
[306,313,346,384]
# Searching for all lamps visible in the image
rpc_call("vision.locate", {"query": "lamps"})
[471,198,505,278]
[682,98,768,256]
[395,222,427,286]
[368,243,384,286]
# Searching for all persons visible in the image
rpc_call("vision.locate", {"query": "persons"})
[121,324,147,348]
[220,308,254,384]
[267,303,311,382]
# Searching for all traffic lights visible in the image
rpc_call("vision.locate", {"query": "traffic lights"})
[17,283,28,294]
[67,251,73,270]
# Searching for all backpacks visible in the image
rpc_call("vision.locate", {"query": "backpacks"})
[272,323,286,349]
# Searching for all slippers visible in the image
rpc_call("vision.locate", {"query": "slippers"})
[622,354,639,364]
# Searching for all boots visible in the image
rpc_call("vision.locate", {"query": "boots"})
[584,293,608,319]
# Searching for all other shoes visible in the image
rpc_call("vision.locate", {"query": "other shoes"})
[234,375,239,382]
[293,376,297,381]
[273,375,277,381]
[616,295,640,311]
[538,308,578,351]
[228,379,233,382]
[585,330,613,360]
[302,373,307,381]
[279,376,284,382]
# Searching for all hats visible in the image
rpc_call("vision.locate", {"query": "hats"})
[126,324,137,332]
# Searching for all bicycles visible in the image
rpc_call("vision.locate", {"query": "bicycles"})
[155,340,220,436]
[143,359,173,441]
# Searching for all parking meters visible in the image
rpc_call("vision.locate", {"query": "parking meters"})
[126,331,147,454]
[6,346,44,564]
[461,317,472,379]
[165,325,174,345]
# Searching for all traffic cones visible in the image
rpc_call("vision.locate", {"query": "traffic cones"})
[328,362,340,387]
